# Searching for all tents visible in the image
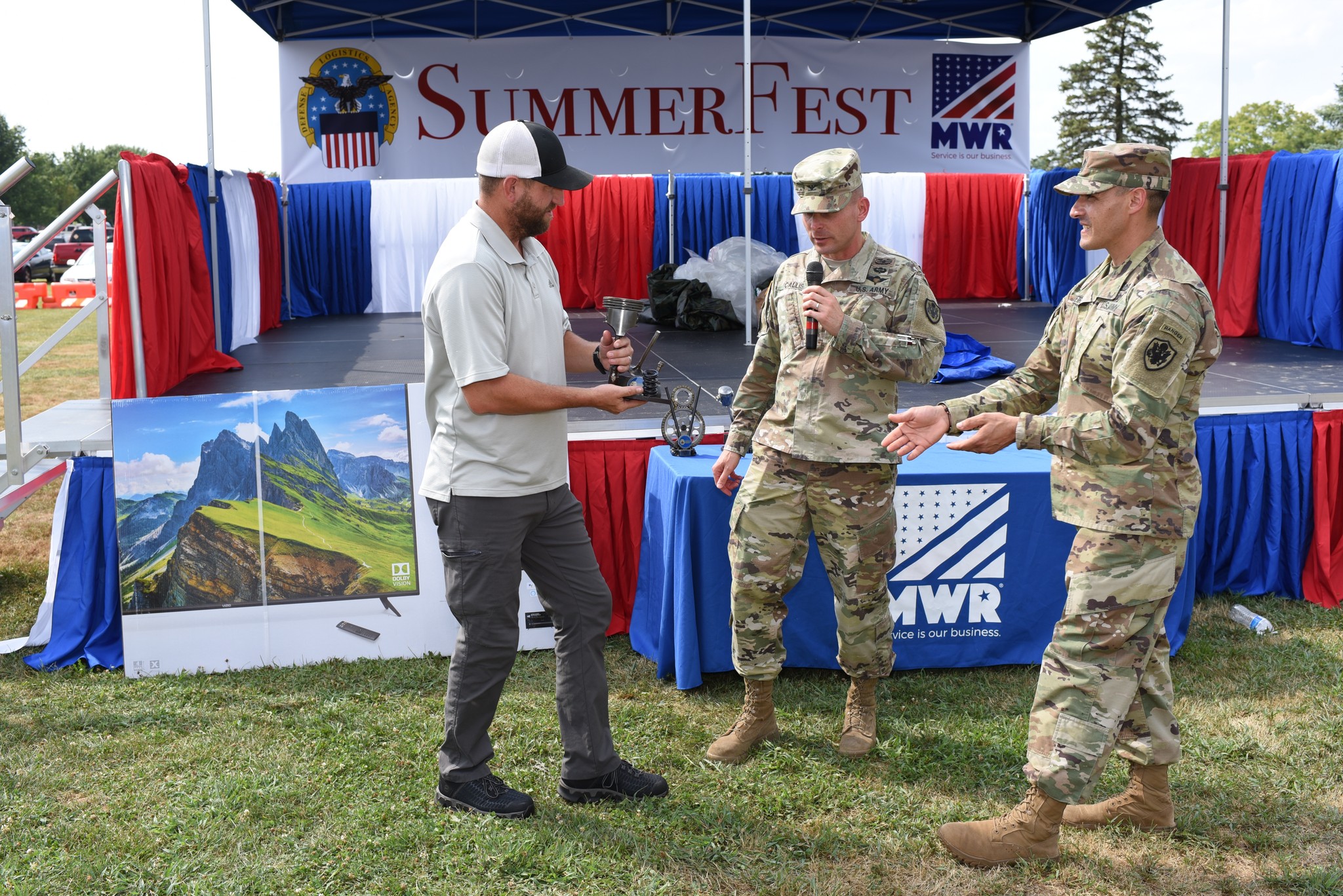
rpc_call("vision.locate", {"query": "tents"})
[202,0,1230,357]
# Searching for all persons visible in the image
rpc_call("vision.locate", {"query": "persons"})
[707,147,947,764]
[879,139,1223,872]
[417,119,669,820]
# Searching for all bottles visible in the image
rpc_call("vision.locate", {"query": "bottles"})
[1228,603,1276,637]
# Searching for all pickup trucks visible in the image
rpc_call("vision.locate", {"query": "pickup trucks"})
[53,227,115,274]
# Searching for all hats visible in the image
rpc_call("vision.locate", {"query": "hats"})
[791,148,863,216]
[1053,142,1173,198]
[475,119,595,191]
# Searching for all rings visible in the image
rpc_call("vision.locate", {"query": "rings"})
[627,356,631,365]
[816,303,819,310]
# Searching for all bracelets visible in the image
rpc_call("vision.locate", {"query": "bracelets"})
[940,404,952,434]
[592,345,610,375]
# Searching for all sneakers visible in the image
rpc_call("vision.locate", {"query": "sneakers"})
[556,757,669,806]
[434,770,535,821]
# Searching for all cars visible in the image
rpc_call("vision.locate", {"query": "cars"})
[12,225,78,251]
[60,242,114,283]
[12,242,56,283]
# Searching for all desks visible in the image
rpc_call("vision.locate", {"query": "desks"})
[628,427,1206,692]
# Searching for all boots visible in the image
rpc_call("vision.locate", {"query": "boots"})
[937,784,1068,870]
[836,676,877,758]
[705,677,781,763]
[1061,762,1177,835]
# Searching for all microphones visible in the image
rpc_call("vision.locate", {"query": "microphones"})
[805,261,824,350]
